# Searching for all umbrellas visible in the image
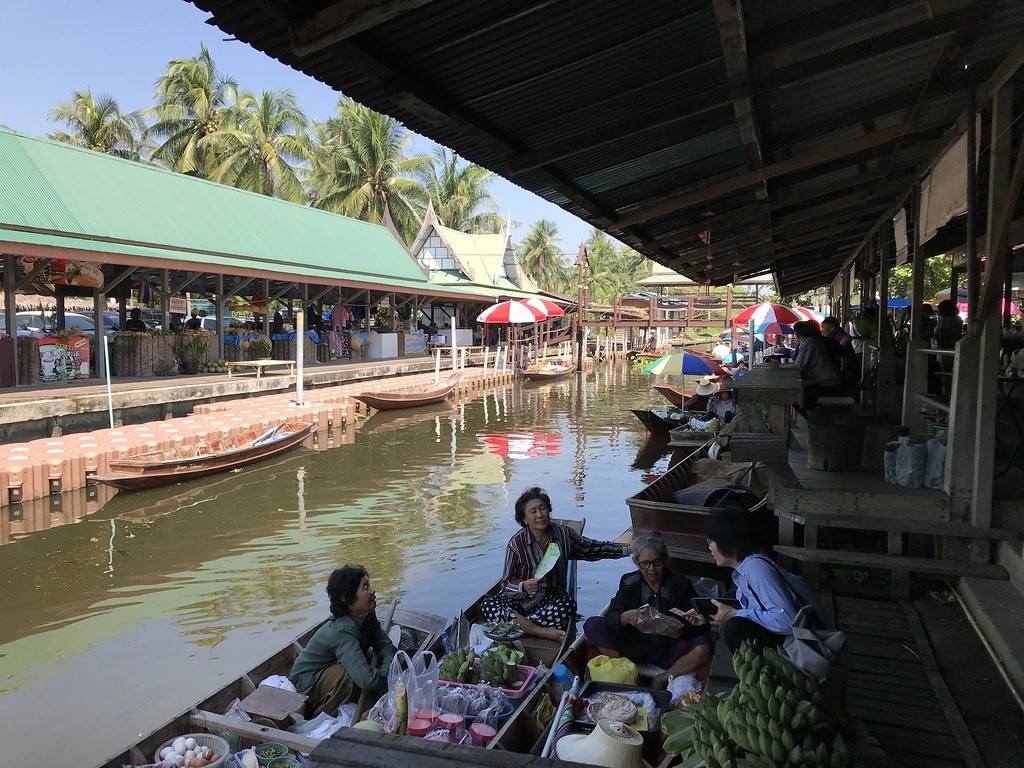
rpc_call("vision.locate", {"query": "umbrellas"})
[936,287,968,301]
[477,299,546,345]
[712,303,825,364]
[642,353,728,413]
[520,298,564,316]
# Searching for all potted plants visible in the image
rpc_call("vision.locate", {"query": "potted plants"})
[252,339,273,370]
[171,335,210,375]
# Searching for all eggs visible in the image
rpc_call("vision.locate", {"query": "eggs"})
[160,737,221,768]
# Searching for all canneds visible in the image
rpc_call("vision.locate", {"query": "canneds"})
[408,709,496,748]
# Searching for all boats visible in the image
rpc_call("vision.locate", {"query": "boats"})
[654,382,699,409]
[627,404,693,438]
[353,369,466,412]
[524,358,576,379]
[101,513,721,768]
[609,419,779,566]
[628,350,665,362]
[83,418,320,487]
[682,347,725,365]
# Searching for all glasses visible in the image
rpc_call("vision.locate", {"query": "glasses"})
[707,538,715,545]
[636,556,664,569]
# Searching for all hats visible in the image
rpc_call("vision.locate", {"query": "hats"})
[554,718,653,768]
[716,384,732,392]
[695,379,718,396]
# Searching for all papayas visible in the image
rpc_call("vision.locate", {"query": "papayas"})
[353,720,385,733]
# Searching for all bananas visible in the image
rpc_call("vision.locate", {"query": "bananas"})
[439,647,525,689]
[691,638,886,768]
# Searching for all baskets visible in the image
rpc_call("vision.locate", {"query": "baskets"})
[254,741,289,764]
[437,656,540,709]
[573,680,672,740]
[154,733,230,768]
[267,757,301,768]
[234,743,307,768]
[437,698,514,729]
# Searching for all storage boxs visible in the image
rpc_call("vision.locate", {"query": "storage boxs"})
[233,750,305,768]
[571,681,672,756]
[436,656,536,696]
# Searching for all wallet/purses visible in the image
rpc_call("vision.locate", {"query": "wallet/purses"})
[691,598,742,614]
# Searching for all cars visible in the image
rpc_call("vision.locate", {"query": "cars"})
[0,313,35,337]
[17,311,114,334]
[78,310,154,331]
[125,308,301,331]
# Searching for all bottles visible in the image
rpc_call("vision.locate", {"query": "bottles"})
[554,664,582,692]
[440,631,454,654]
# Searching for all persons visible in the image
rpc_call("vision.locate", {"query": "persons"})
[289,566,394,716]
[734,361,749,379]
[776,318,861,421]
[307,306,320,325]
[584,534,708,689]
[480,488,632,643]
[683,508,820,653]
[923,301,963,390]
[126,308,146,332]
[169,313,201,331]
[684,379,717,411]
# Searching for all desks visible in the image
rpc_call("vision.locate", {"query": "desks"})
[726,361,804,490]
[936,371,1024,474]
[227,360,296,378]
[431,346,485,358]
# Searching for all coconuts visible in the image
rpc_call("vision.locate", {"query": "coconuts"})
[704,418,726,432]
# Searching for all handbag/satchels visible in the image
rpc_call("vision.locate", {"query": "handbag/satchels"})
[895,440,925,488]
[781,604,848,687]
[667,675,701,705]
[386,649,437,734]
[925,437,946,488]
[884,442,897,485]
[518,591,548,612]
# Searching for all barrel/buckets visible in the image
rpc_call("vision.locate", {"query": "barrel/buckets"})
[434,693,513,730]
